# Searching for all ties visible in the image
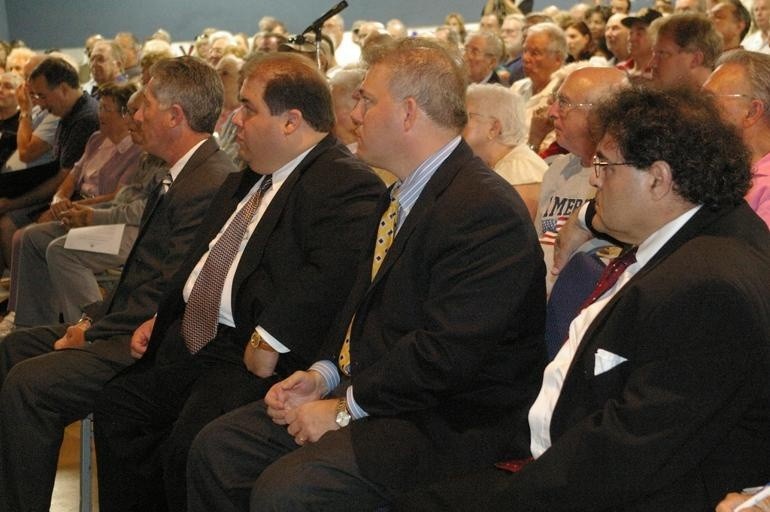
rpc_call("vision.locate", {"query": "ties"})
[339,187,402,378]
[178,174,273,355]
[492,248,637,471]
[141,173,173,226]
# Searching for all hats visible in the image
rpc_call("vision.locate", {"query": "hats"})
[279,33,330,66]
[353,21,366,32]
[622,7,664,27]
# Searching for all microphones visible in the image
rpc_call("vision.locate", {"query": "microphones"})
[289,1,349,46]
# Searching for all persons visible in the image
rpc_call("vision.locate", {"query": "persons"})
[708,1,750,52]
[278,32,337,71]
[647,11,723,88]
[740,0,770,54]
[701,50,770,230]
[91,42,128,85]
[321,14,360,64]
[657,1,717,17]
[582,6,612,64]
[0,40,34,70]
[478,0,532,30]
[1,55,61,198]
[564,21,592,62]
[85,36,102,58]
[1,72,23,166]
[716,485,770,512]
[529,62,569,164]
[6,83,138,313]
[462,84,548,218]
[497,15,524,85]
[8,88,164,328]
[535,66,628,295]
[140,41,172,83]
[512,25,566,102]
[94,58,391,512]
[526,4,589,27]
[194,17,286,61]
[116,32,142,78]
[152,30,170,44]
[214,54,246,168]
[464,33,502,85]
[324,61,398,186]
[615,7,661,75]
[185,38,548,512]
[608,0,631,14]
[398,75,770,511]
[2,58,239,509]
[433,13,466,52]
[350,17,409,43]
[605,13,633,65]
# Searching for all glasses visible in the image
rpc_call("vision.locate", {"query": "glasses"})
[468,111,487,125]
[593,152,654,177]
[555,93,598,113]
[194,34,210,41]
[289,35,315,45]
[463,47,492,59]
[711,91,753,103]
[31,91,62,105]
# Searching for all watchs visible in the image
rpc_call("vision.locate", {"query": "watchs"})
[77,314,93,323]
[19,112,33,121]
[250,331,276,354]
[337,397,350,427]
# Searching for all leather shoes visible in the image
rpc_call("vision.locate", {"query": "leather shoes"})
[1,311,18,338]
